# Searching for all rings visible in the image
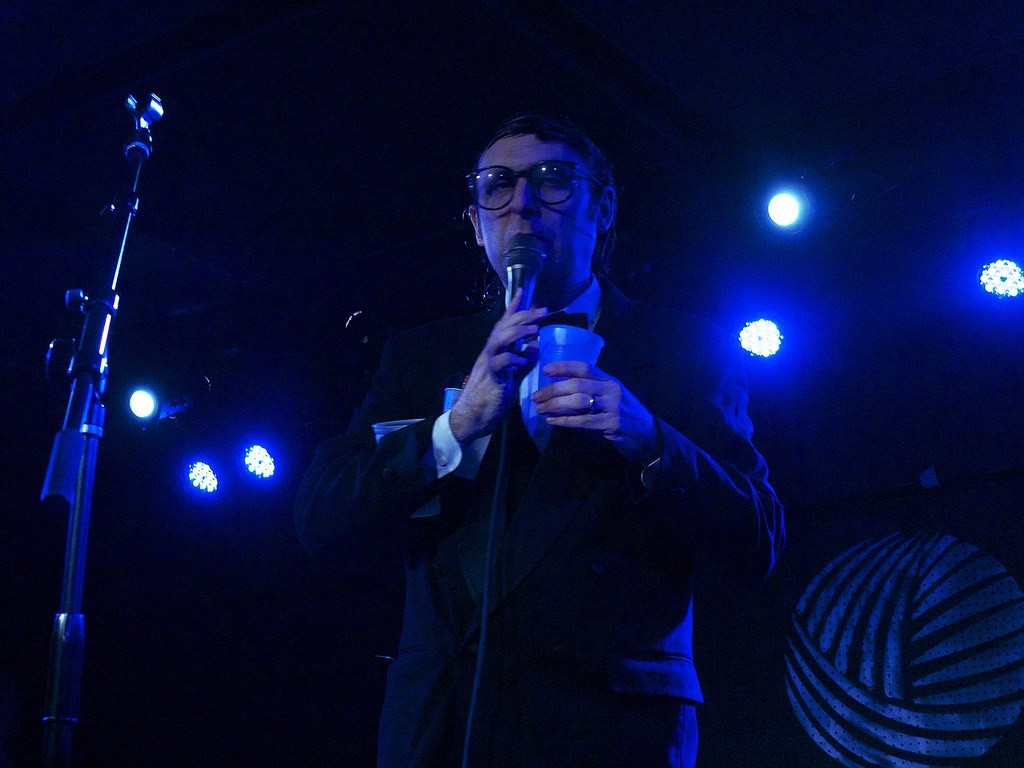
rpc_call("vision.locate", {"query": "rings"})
[590,397,597,409]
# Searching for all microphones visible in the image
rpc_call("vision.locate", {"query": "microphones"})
[503,233,548,373]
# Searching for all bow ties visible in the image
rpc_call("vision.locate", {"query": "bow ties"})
[524,311,588,344]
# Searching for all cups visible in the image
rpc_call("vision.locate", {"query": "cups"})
[370,418,441,519]
[441,388,495,480]
[537,324,605,389]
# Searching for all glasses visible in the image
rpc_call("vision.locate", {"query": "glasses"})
[466,159,603,210]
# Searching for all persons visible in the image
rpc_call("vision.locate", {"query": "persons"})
[293,109,787,768]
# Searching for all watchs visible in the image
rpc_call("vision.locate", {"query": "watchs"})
[638,454,681,499]
